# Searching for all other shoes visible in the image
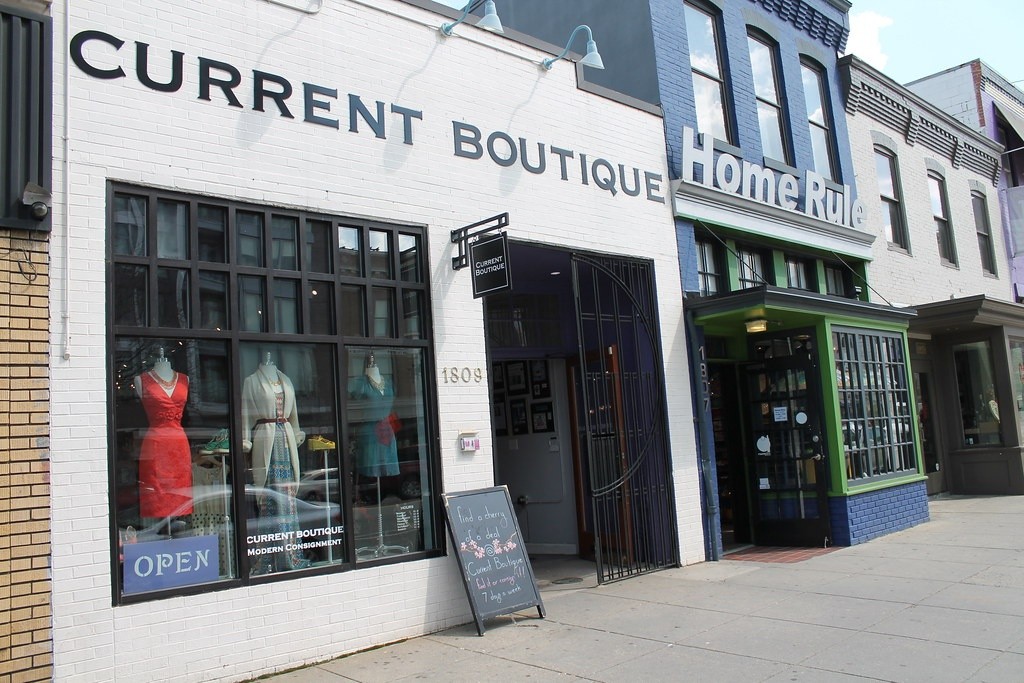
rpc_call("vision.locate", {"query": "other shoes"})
[307,434,335,451]
[118,526,137,563]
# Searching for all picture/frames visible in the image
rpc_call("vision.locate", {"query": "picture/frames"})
[528,358,552,400]
[509,398,529,436]
[492,363,504,390]
[505,360,530,396]
[529,401,555,433]
[494,399,509,437]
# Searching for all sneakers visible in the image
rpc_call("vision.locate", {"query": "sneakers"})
[198,428,230,454]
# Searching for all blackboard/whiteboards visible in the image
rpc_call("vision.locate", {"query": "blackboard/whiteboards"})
[442,484,541,621]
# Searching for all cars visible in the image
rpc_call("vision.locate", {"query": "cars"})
[358,444,429,506]
[117,484,341,563]
[266,468,353,502]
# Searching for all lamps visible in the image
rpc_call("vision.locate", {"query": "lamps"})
[542,23,605,72]
[442,0,507,37]
[745,319,768,332]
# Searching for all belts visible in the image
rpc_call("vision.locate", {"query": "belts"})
[256,418,290,424]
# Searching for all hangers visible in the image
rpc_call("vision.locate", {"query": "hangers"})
[191,444,222,467]
